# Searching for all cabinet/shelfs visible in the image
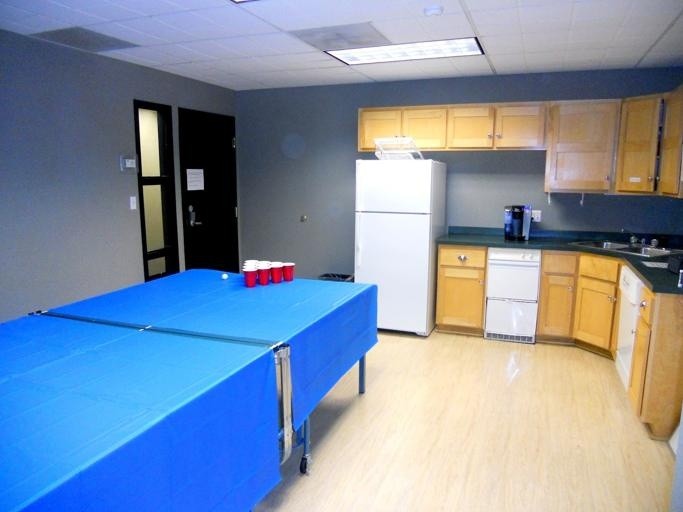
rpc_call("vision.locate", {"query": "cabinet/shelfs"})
[447,105,545,149]
[573,253,620,352]
[536,249,580,347]
[435,244,487,337]
[615,91,683,195]
[628,288,657,417]
[360,108,447,149]
[548,102,616,190]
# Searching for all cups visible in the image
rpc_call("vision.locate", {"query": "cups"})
[241,257,296,288]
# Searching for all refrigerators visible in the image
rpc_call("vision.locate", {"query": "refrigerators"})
[353,158,447,338]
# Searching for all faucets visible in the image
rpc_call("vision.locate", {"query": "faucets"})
[617,224,647,247]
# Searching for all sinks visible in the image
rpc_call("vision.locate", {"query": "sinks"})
[567,238,639,251]
[613,245,672,258]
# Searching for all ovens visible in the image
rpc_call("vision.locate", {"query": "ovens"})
[483,247,540,344]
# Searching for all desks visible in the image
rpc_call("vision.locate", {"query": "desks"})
[0,269,379,512]
